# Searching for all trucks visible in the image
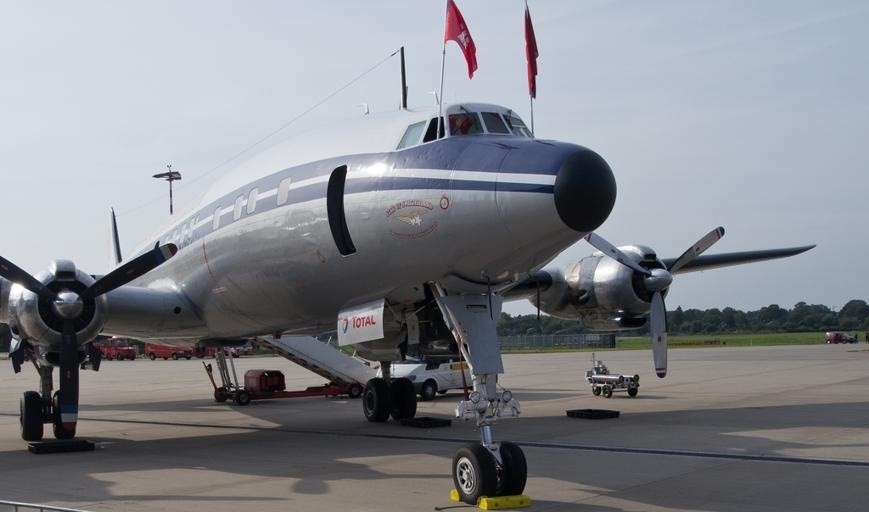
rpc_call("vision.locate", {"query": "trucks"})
[585,362,641,398]
[94,335,135,361]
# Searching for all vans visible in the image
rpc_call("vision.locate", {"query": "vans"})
[144,338,193,360]
[374,359,473,400]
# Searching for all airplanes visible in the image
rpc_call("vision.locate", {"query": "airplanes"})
[0,100,816,505]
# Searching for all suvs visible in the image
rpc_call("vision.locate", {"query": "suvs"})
[826,331,854,344]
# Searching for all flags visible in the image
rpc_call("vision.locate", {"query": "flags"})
[523,4,538,99]
[444,0,478,80]
